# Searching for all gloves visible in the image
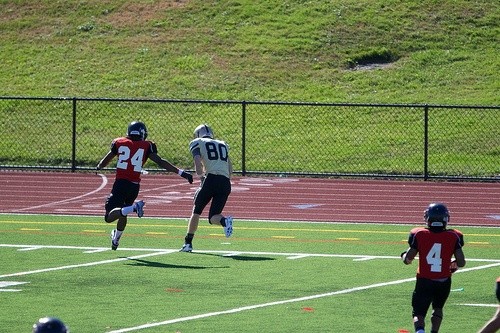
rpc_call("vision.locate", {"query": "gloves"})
[181,171,193,184]
[96,163,101,171]
[450,260,458,273]
[401,251,406,258]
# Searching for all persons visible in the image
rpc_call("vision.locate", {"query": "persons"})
[400,204,466,333]
[96,120,193,252]
[479,276,500,333]
[179,124,233,253]
[31,317,68,333]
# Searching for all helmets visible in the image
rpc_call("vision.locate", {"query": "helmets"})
[193,123,214,139]
[126,121,147,141]
[33,317,68,333]
[424,202,450,232]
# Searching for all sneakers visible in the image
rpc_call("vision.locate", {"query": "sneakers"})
[134,200,145,218]
[224,216,233,237]
[179,244,192,252]
[111,229,119,250]
